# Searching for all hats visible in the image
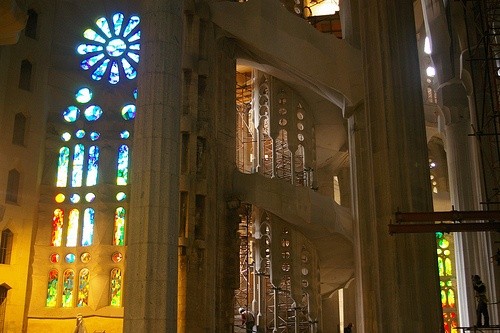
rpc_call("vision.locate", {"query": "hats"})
[238,307,244,313]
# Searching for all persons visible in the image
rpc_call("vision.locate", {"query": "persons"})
[239,308,256,333]
[344,323,356,333]
[471,275,490,327]
